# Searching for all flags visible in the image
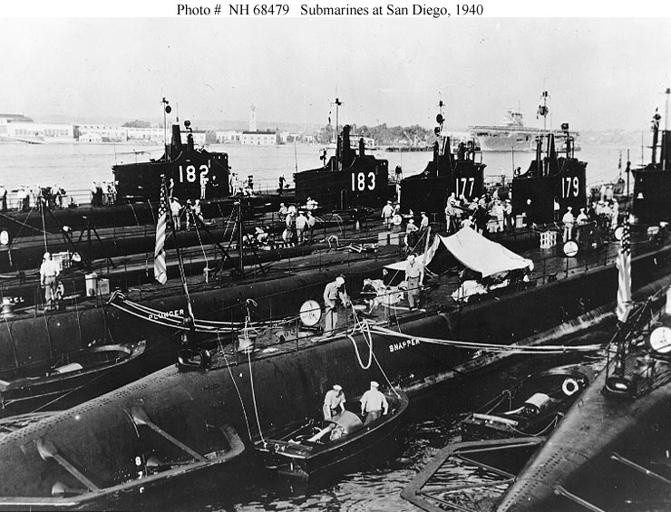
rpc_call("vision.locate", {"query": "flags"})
[614,213,635,324]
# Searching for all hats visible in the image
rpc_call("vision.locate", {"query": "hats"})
[505,199,511,202]
[44,253,49,259]
[388,201,392,204]
[409,212,425,222]
[285,211,312,214]
[371,381,380,388]
[336,277,345,283]
[568,206,584,211]
[333,385,342,391]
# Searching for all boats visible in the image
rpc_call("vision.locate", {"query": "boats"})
[0,90,671,511]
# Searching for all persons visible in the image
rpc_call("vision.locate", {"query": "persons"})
[360,379,390,427]
[444,180,623,246]
[325,384,348,421]
[0,167,435,344]
